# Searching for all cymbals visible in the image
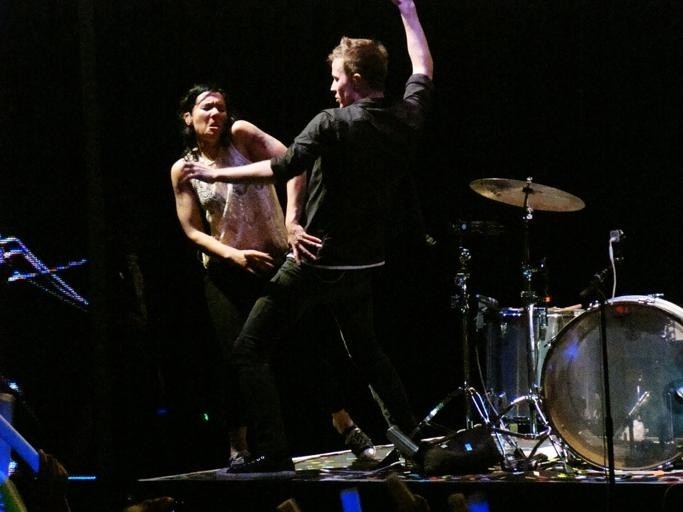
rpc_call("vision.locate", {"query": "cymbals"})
[469,177,585,212]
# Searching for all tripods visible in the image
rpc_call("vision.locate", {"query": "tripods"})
[375,192,586,479]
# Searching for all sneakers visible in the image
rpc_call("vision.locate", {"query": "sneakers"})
[339,420,376,459]
[215,451,296,480]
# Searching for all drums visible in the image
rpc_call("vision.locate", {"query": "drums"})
[540,292,683,470]
[486,304,586,427]
[545,305,586,340]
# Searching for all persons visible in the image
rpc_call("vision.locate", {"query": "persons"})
[171,83,377,472]
[178,0,434,476]
